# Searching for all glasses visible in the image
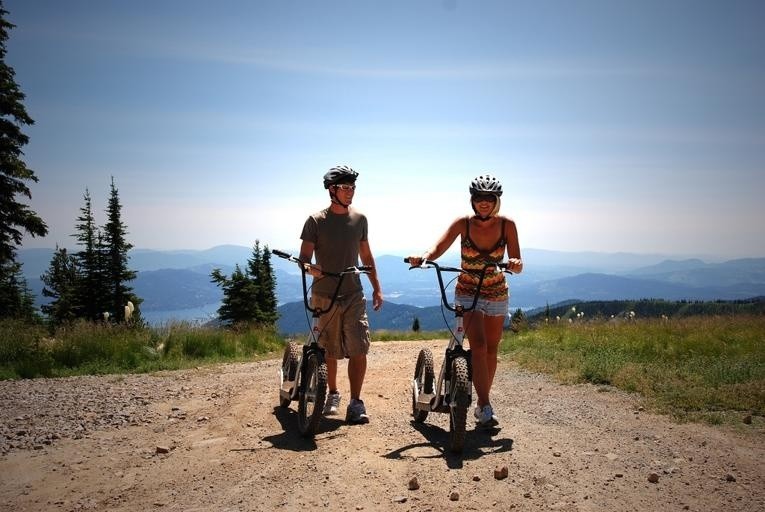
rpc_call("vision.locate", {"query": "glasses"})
[331,184,356,191]
[472,194,496,203]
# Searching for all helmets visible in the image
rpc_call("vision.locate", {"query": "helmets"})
[470,175,503,196]
[323,165,359,188]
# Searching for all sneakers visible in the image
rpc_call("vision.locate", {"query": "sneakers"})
[478,404,498,427]
[323,392,341,414]
[346,398,369,424]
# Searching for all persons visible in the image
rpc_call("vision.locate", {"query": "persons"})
[407,173,523,426]
[298,164,383,422]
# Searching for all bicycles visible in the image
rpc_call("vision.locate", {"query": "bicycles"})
[398,253,517,457]
[266,243,377,441]
